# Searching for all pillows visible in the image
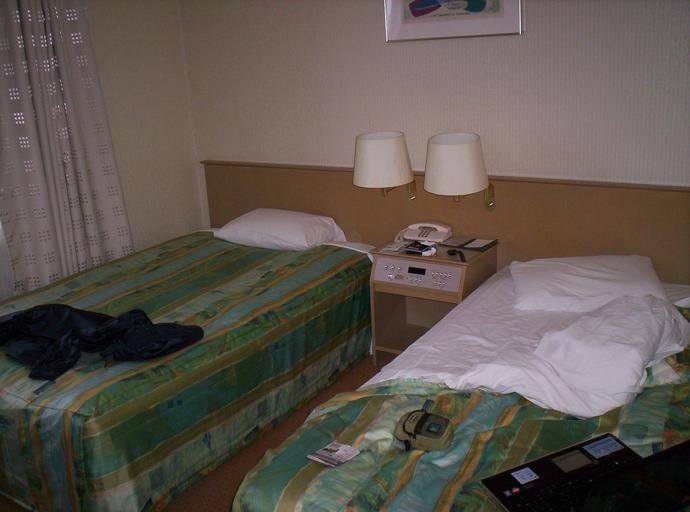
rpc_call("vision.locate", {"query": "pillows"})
[211,206,346,254]
[506,250,677,396]
[231,257,690,512]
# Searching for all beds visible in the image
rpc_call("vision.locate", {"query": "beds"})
[0,227,379,512]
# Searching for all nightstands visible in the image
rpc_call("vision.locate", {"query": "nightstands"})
[368,237,501,365]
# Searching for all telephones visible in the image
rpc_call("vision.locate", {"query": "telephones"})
[401,223,453,244]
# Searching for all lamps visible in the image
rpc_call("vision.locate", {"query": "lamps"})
[351,129,496,213]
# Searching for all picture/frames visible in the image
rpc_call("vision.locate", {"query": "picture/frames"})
[381,1,526,43]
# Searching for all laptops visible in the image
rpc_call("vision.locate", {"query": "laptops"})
[481,433,690,512]
